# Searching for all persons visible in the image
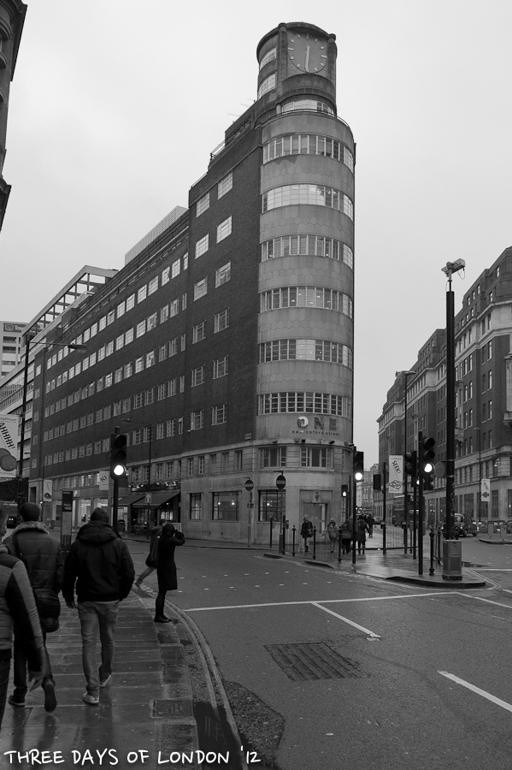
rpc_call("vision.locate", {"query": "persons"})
[320,511,377,555]
[299,514,314,552]
[3,501,63,709]
[61,507,137,708]
[0,514,19,542]
[0,502,49,734]
[148,522,187,626]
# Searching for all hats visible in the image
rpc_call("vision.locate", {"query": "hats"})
[21,502,41,517]
[89,505,110,523]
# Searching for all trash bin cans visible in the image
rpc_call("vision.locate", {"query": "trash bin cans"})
[442,540,463,581]
[117,520,125,537]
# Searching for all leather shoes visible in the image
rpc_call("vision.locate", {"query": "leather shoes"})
[153,615,172,623]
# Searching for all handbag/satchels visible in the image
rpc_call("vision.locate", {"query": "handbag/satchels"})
[145,539,160,568]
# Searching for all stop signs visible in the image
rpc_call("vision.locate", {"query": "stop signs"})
[276,475,286,490]
[244,480,254,491]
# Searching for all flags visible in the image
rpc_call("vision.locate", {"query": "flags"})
[0,413,21,479]
[480,478,492,503]
[387,454,406,495]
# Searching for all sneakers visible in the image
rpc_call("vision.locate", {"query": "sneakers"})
[99,670,112,687]
[43,679,58,712]
[81,691,100,705]
[9,694,26,707]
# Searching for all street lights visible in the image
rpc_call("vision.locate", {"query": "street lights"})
[16,334,87,504]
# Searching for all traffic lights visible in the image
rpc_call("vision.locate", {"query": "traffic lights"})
[109,432,129,481]
[423,436,435,490]
[354,451,365,482]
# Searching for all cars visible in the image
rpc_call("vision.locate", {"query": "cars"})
[441,512,479,540]
